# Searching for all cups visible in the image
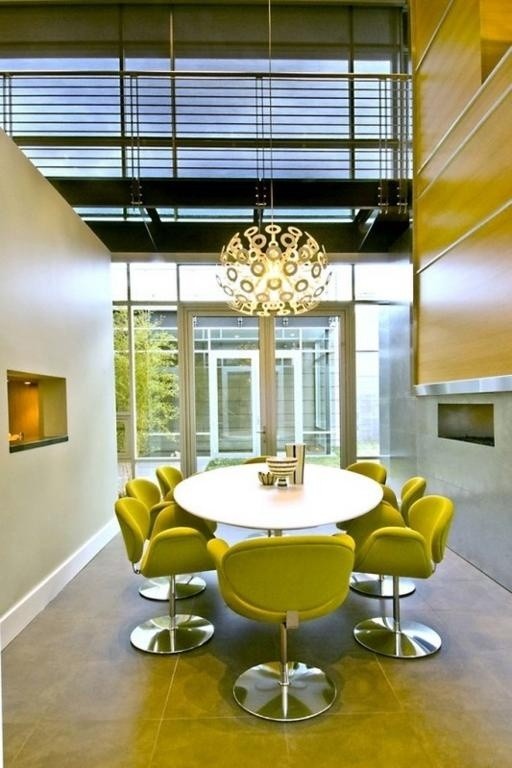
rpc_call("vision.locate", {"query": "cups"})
[285,444,306,485]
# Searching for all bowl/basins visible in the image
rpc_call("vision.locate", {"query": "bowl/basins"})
[258,472,278,485]
[266,456,298,486]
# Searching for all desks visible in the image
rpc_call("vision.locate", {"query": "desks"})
[172,461,384,537]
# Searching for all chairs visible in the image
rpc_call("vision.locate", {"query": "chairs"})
[206,533,356,722]
[114,496,217,655]
[156,465,204,498]
[125,477,217,600]
[336,476,427,597]
[346,461,387,484]
[346,494,455,659]
[241,455,272,463]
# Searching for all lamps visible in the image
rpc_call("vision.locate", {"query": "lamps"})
[216,0,333,316]
